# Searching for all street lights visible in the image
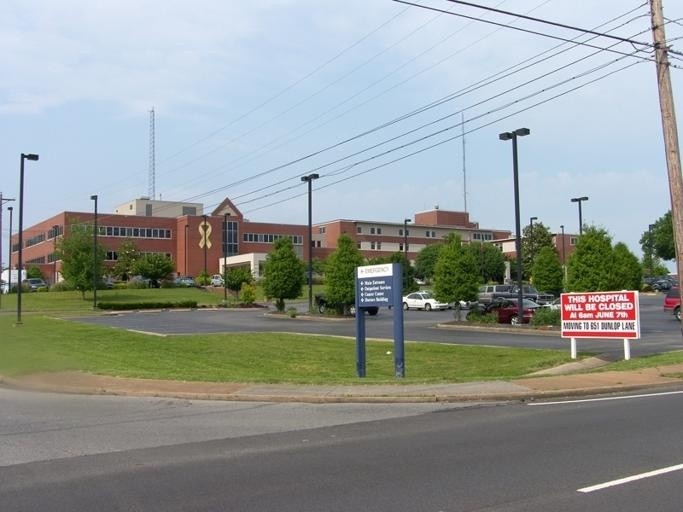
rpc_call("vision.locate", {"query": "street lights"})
[530,217,538,283]
[201,214,207,286]
[558,225,565,284]
[301,173,319,313]
[184,225,190,277]
[500,127,530,323]
[570,196,588,237]
[648,223,655,276]
[91,194,97,308]
[7,207,13,294]
[404,219,412,288]
[52,225,58,284]
[225,212,232,300]
[18,152,39,324]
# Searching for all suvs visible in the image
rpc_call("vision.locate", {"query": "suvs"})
[663,285,681,322]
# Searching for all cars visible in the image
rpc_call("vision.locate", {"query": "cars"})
[314,290,379,318]
[641,274,680,292]
[465,298,550,326]
[403,284,562,311]
[302,270,327,285]
[73,274,226,291]
[14,278,49,293]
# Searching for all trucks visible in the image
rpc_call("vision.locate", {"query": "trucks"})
[1,269,26,294]
